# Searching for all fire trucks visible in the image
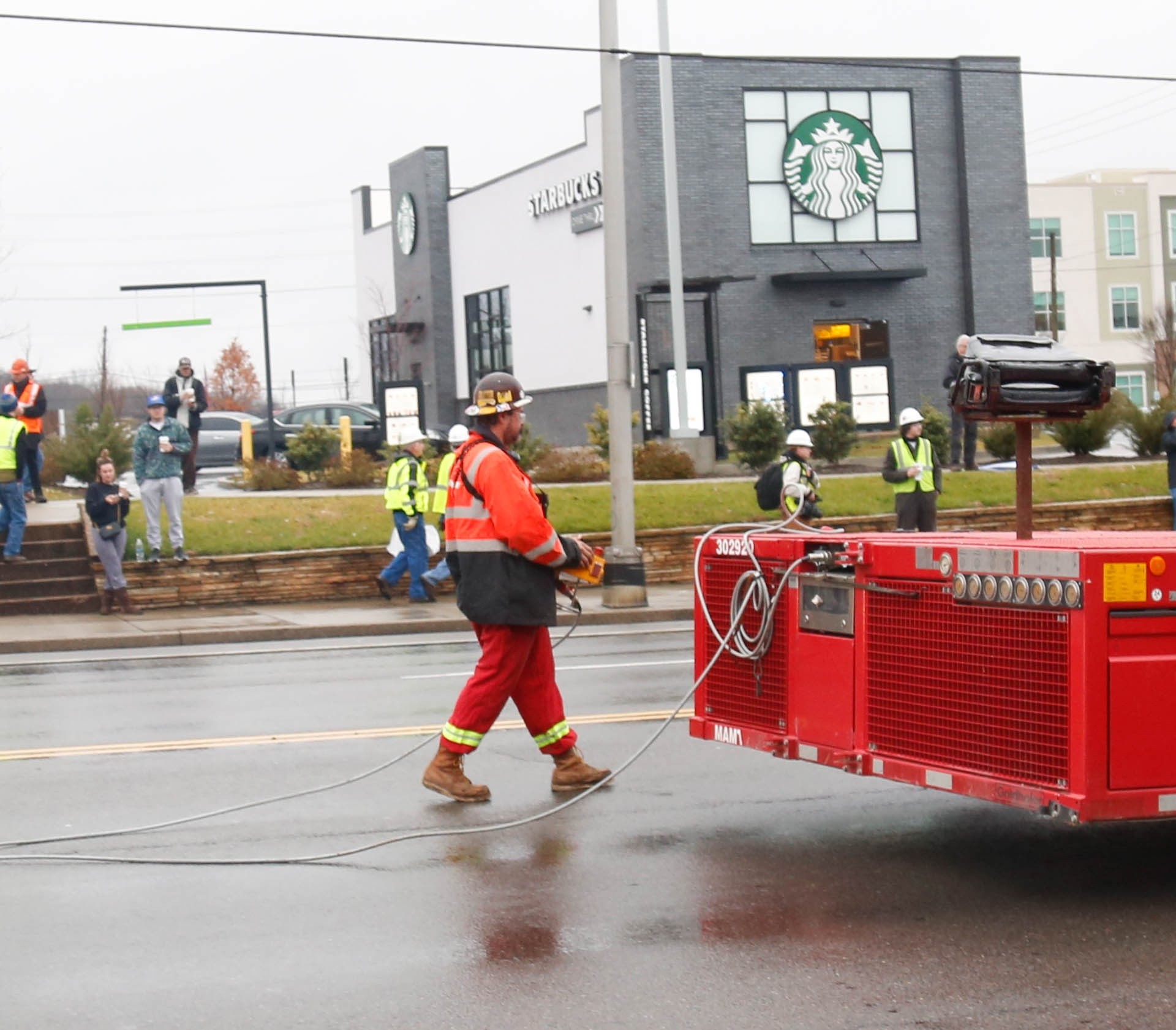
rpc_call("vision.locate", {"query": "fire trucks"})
[691,332,1174,832]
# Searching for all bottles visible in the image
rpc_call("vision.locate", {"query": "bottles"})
[136,538,145,562]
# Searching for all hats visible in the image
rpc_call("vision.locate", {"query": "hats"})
[179,357,191,369]
[147,394,166,407]
[0,393,19,412]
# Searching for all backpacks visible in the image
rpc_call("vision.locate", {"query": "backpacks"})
[753,458,809,511]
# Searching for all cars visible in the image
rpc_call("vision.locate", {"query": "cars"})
[233,401,446,467]
[195,411,266,471]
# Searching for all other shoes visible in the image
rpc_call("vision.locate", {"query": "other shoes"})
[174,547,189,562]
[34,494,46,502]
[551,748,615,793]
[410,597,437,603]
[4,555,26,562]
[422,751,490,801]
[419,576,434,601]
[376,575,392,600]
[148,549,161,563]
[950,465,979,471]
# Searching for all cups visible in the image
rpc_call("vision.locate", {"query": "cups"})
[118,481,126,497]
[187,388,196,403]
[159,436,170,453]
[914,462,924,481]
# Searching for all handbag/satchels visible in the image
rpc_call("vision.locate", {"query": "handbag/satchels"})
[386,525,441,558]
[100,522,120,540]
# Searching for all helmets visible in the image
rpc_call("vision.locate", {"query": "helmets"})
[447,423,469,443]
[399,426,428,446]
[464,373,534,416]
[11,360,29,374]
[785,429,814,447]
[899,407,924,426]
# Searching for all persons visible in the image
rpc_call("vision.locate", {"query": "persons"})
[4,357,48,504]
[134,394,195,563]
[374,427,436,603]
[21,439,44,500]
[0,393,29,563]
[420,423,470,589]
[422,372,614,802]
[881,407,942,532]
[942,333,980,472]
[778,429,822,529]
[1162,410,1176,530]
[163,356,208,496]
[85,448,141,616]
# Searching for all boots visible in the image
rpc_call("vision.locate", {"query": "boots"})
[115,587,141,614]
[99,589,112,615]
